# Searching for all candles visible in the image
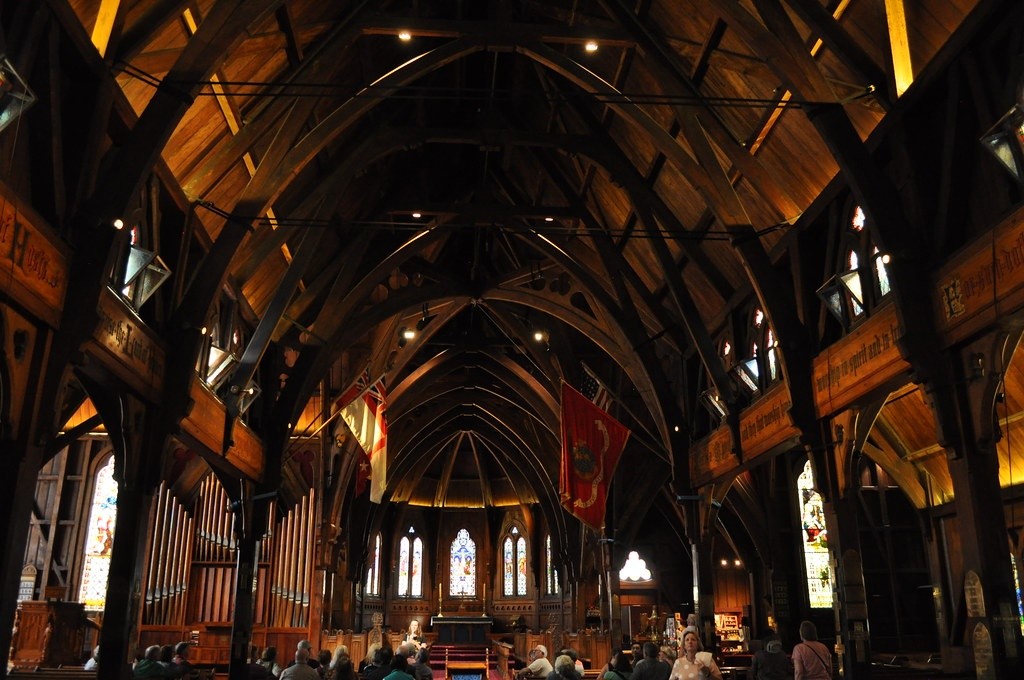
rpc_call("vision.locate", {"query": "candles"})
[439,583,442,598]
[483,582,486,599]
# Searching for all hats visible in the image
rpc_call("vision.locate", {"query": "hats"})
[175,642,191,654]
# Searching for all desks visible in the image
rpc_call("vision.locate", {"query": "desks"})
[431,615,494,643]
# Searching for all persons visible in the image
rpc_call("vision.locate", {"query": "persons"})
[130,640,199,679]
[513,612,725,680]
[84,645,100,670]
[251,618,433,680]
[792,621,833,680]
[751,628,791,680]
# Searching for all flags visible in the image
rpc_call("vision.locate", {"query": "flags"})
[554,379,632,537]
[336,360,393,504]
